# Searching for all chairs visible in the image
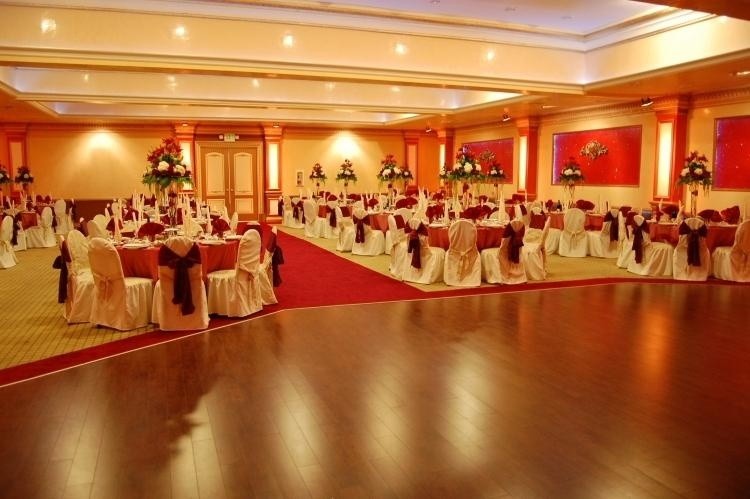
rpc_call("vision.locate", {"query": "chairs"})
[0,191,75,270]
[50,196,278,332]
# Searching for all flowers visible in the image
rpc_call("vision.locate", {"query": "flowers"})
[376,154,401,181]
[556,158,584,181]
[141,136,193,190]
[483,160,509,181]
[336,159,358,182]
[397,165,414,180]
[677,151,713,186]
[437,165,452,179]
[452,146,484,183]
[310,163,326,181]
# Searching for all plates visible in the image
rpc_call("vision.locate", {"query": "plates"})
[105,234,242,250]
